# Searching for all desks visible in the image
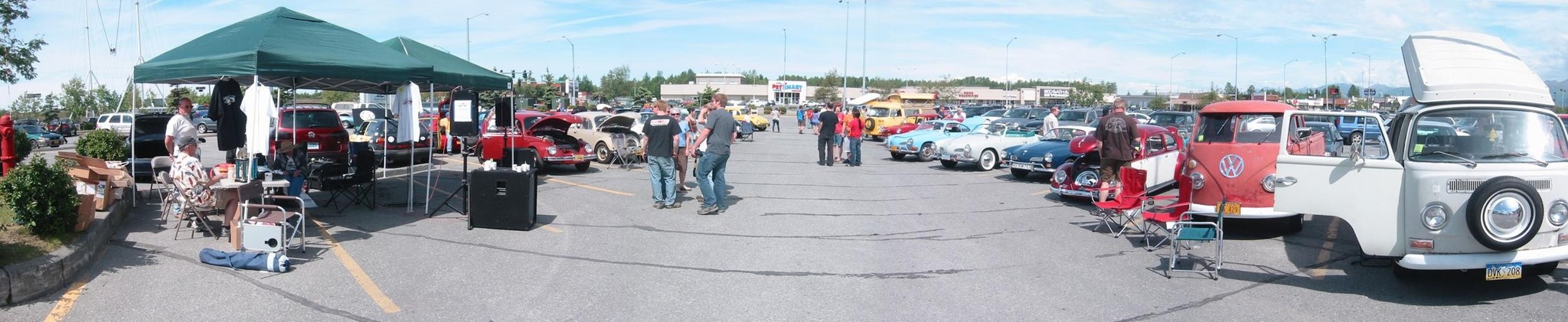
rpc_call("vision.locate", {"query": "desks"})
[208,166,290,191]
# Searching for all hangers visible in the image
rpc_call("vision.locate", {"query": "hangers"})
[219,75,232,82]
[403,79,413,87]
[248,79,263,87]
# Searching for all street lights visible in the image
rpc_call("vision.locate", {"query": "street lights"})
[1169,52,1185,111]
[466,13,489,63]
[1283,59,1297,104]
[1312,33,1338,110]
[783,28,785,108]
[897,67,916,92]
[838,0,849,111]
[1217,34,1237,101]
[562,36,575,106]
[862,0,866,96]
[433,45,449,54]
[546,39,571,81]
[1351,51,1370,110]
[1005,37,1017,110]
[1061,71,1077,108]
[714,63,735,84]
[742,69,762,99]
[1361,68,1374,98]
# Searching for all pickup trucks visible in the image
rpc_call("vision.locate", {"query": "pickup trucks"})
[337,103,384,129]
[802,101,820,108]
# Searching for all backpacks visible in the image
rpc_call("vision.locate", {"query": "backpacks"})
[798,110,803,121]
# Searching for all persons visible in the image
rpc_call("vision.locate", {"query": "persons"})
[53,117,67,144]
[274,140,309,208]
[1042,107,1059,139]
[226,134,247,164]
[770,108,781,132]
[1543,118,1555,155]
[164,97,197,220]
[603,107,616,114]
[745,110,750,123]
[797,102,867,167]
[938,105,945,118]
[169,136,239,237]
[36,118,45,129]
[1090,97,1140,216]
[556,103,564,112]
[953,107,966,120]
[440,112,463,155]
[639,94,738,214]
[578,102,587,112]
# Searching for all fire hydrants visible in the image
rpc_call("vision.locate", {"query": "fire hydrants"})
[0,113,19,178]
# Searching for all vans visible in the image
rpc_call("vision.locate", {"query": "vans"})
[1272,30,1568,281]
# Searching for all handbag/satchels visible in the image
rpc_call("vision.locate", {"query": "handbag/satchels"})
[693,134,699,140]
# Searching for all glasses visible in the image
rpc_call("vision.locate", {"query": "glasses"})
[181,104,193,109]
[671,113,680,115]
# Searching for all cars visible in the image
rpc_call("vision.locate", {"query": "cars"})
[477,111,491,132]
[349,119,436,167]
[80,116,98,131]
[845,93,1568,140]
[1048,124,1188,201]
[935,122,1044,172]
[126,113,206,177]
[418,98,780,164]
[190,105,217,133]
[48,118,77,137]
[12,119,65,149]
[884,115,991,162]
[1000,125,1098,180]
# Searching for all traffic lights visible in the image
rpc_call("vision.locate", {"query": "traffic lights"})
[512,70,514,79]
[522,71,526,79]
[518,79,521,85]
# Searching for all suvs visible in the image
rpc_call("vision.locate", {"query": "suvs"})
[95,112,144,137]
[269,102,349,162]
[331,101,358,117]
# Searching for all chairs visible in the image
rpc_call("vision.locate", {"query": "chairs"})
[1139,172,1193,250]
[605,132,643,171]
[172,178,221,241]
[740,121,754,142]
[147,154,172,205]
[236,179,306,259]
[209,162,234,179]
[348,141,375,174]
[320,148,377,213]
[1084,164,1147,239]
[1166,193,1227,282]
[156,170,194,229]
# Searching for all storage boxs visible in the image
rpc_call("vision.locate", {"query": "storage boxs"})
[55,148,135,233]
[230,219,276,251]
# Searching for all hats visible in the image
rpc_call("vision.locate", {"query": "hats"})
[177,135,200,147]
[277,140,299,153]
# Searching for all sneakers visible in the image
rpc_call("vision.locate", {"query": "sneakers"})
[1091,210,1105,216]
[696,195,724,214]
[175,214,190,219]
[676,185,681,192]
[835,158,841,161]
[191,221,214,232]
[447,152,452,155]
[1110,210,1124,217]
[221,226,230,237]
[679,185,691,190]
[654,202,680,208]
